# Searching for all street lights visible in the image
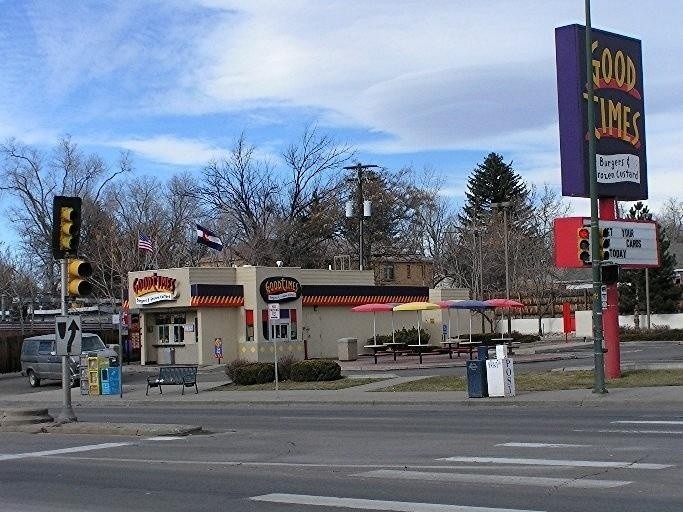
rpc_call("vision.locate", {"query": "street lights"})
[488,201,512,337]
[342,162,379,269]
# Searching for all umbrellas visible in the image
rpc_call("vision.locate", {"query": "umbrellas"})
[451,299,486,341]
[435,300,456,340]
[350,302,391,345]
[484,298,524,339]
[392,301,439,344]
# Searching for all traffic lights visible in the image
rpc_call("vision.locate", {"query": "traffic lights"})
[51,195,83,260]
[600,227,611,261]
[67,258,93,298]
[576,227,592,263]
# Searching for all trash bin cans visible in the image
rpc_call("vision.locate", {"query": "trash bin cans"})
[337,338,357,362]
[466,344,515,398]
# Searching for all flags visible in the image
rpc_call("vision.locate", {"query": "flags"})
[195,223,223,252]
[137,231,152,251]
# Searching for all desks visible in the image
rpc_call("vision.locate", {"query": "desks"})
[362,337,513,364]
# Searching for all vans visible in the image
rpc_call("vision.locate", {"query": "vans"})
[19,331,119,389]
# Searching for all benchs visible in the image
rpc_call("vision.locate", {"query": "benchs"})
[358,347,520,363]
[145,366,200,398]
